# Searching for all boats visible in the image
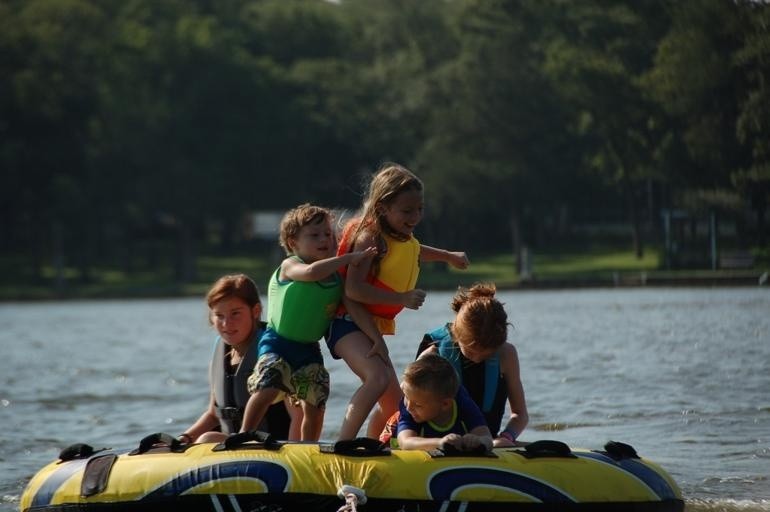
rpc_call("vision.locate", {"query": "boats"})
[19,429,685,512]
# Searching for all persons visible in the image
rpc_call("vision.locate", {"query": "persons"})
[239,204,389,441]
[324,165,470,441]
